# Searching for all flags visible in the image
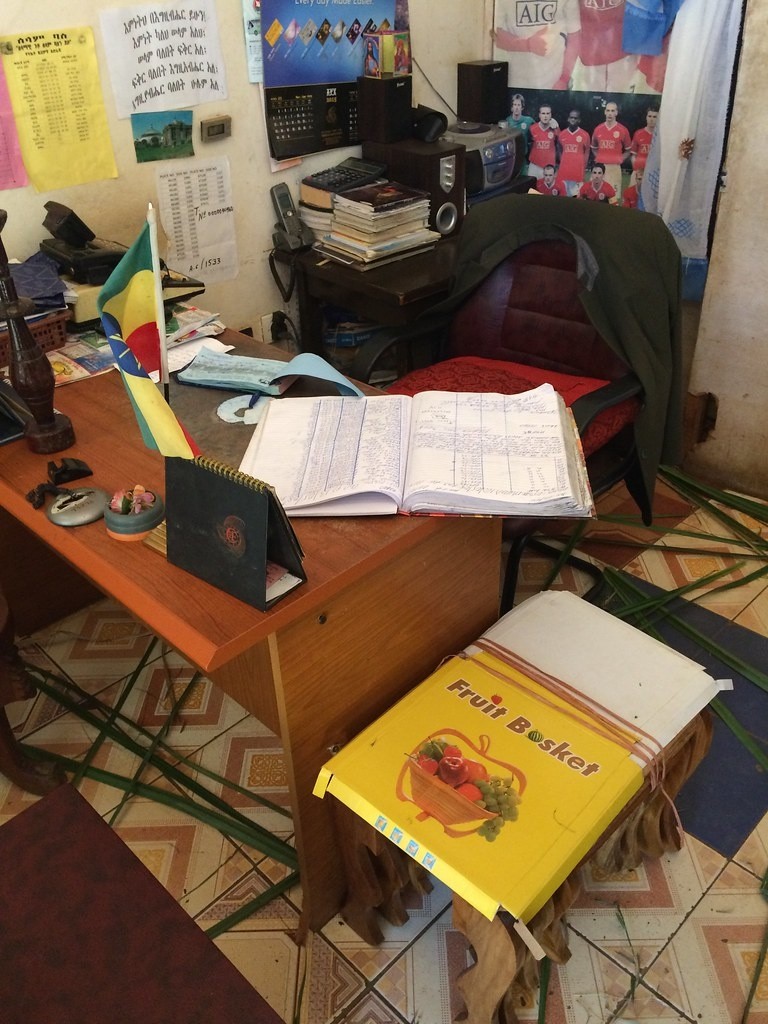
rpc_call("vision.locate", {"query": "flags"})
[96,209,204,461]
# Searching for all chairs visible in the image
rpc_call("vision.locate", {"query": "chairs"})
[347,192,684,618]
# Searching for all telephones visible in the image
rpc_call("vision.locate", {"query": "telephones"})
[270,183,315,253]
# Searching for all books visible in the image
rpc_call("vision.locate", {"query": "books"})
[299,170,441,273]
[176,344,365,398]
[238,383,599,520]
[0,331,116,387]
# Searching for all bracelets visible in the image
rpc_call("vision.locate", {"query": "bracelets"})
[560,75,571,83]
[526,38,531,52]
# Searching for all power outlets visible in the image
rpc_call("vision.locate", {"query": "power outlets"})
[260,311,289,343]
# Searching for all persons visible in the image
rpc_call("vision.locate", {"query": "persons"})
[504,94,561,181]
[558,107,590,197]
[535,164,566,197]
[623,169,644,209]
[489,0,685,96]
[394,39,408,75]
[591,101,632,201]
[578,162,619,206]
[364,41,379,77]
[629,107,659,189]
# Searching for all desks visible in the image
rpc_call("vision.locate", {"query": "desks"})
[0,328,512,950]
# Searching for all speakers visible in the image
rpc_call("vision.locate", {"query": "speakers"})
[356,71,413,143]
[456,59,513,123]
[362,137,466,240]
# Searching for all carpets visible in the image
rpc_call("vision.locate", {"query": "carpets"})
[0,781,282,1024]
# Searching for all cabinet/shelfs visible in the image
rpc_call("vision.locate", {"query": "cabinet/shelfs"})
[273,245,452,389]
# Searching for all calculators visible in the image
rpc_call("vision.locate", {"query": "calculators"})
[301,156,389,194]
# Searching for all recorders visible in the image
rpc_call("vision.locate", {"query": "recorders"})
[438,121,526,198]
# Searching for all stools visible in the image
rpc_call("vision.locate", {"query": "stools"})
[312,587,734,1024]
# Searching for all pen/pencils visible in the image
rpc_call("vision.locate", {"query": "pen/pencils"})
[249,390,263,408]
